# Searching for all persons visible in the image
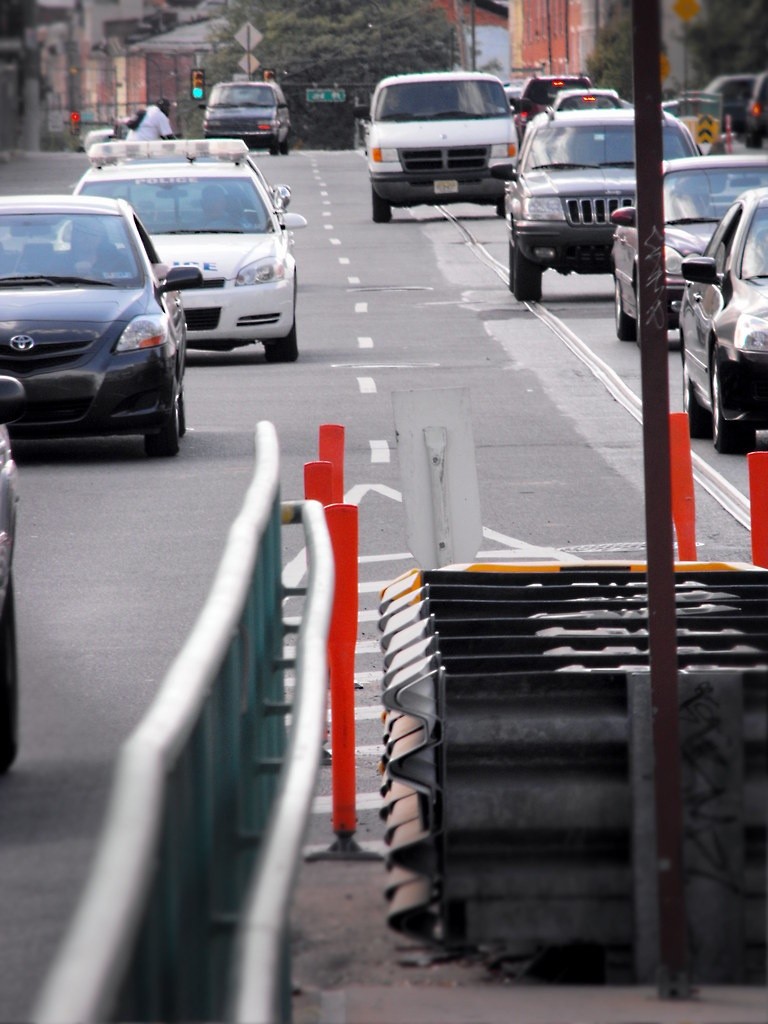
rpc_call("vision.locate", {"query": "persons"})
[68,219,128,276]
[196,185,242,226]
[126,99,177,141]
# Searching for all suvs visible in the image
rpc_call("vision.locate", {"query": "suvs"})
[52,136,309,365]
[501,102,714,302]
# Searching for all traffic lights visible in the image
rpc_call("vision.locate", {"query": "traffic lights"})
[71,111,81,135]
[190,69,206,100]
[262,67,276,82]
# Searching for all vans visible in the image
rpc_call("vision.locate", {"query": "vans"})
[352,70,534,224]
[197,79,293,156]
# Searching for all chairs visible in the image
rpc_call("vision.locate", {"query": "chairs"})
[82,239,124,275]
[255,92,271,102]
[227,194,246,221]
[221,92,235,104]
[11,241,56,273]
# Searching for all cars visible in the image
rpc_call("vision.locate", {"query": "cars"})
[609,151,768,356]
[676,184,768,457]
[500,70,768,165]
[0,191,205,461]
